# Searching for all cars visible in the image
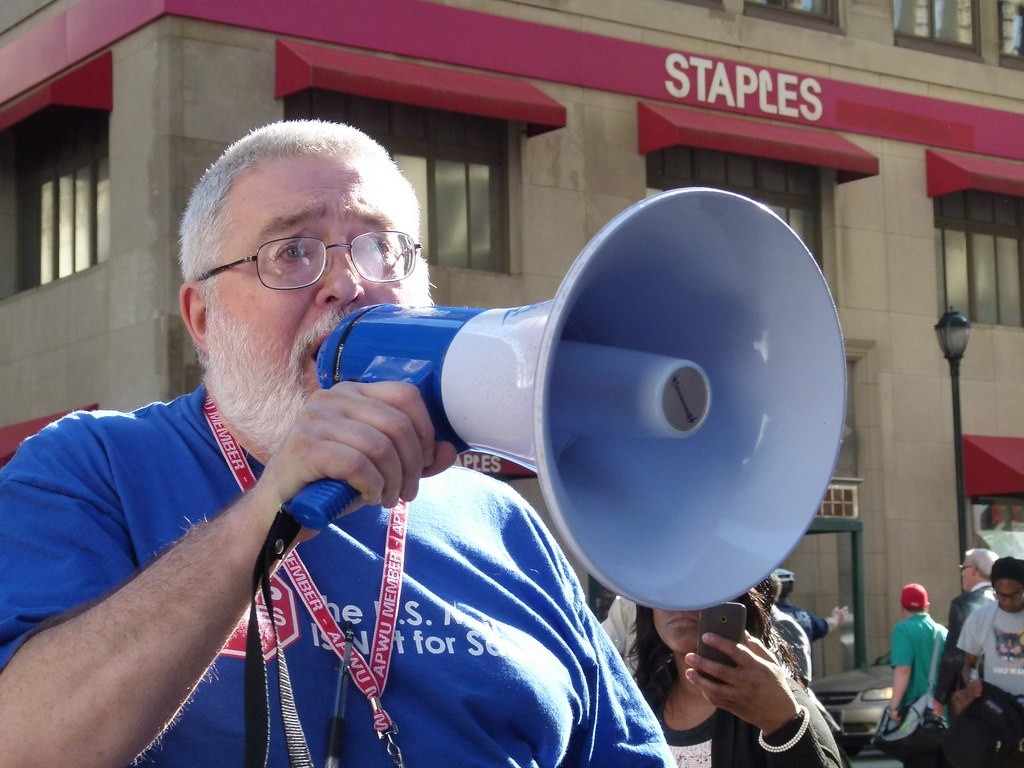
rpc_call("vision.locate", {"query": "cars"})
[807,648,895,758]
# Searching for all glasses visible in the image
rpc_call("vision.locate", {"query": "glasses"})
[196,230,422,290]
[959,565,973,572]
[993,594,1022,600]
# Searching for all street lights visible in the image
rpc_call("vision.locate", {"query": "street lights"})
[934,307,972,595]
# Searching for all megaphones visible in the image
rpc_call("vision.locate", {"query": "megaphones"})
[282,187,848,610]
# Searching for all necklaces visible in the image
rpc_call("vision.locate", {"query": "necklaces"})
[205,397,408,738]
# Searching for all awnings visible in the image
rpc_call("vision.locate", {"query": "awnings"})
[926,150,1024,201]
[0,55,112,133]
[276,38,566,138]
[0,403,99,468]
[961,434,1024,496]
[637,100,881,184]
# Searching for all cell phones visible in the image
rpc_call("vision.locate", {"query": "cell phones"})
[697,601,747,684]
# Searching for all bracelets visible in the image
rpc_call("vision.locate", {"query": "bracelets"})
[890,708,898,711]
[757,707,810,753]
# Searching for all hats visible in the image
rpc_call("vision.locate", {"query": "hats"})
[900,583,928,608]
[774,568,794,581]
[991,557,1024,587]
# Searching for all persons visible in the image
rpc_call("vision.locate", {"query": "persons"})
[0,119,680,768]
[601,567,850,768]
[887,547,1024,768]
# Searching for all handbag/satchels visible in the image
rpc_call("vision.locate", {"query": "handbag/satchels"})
[873,706,948,768]
[943,682,1024,768]
